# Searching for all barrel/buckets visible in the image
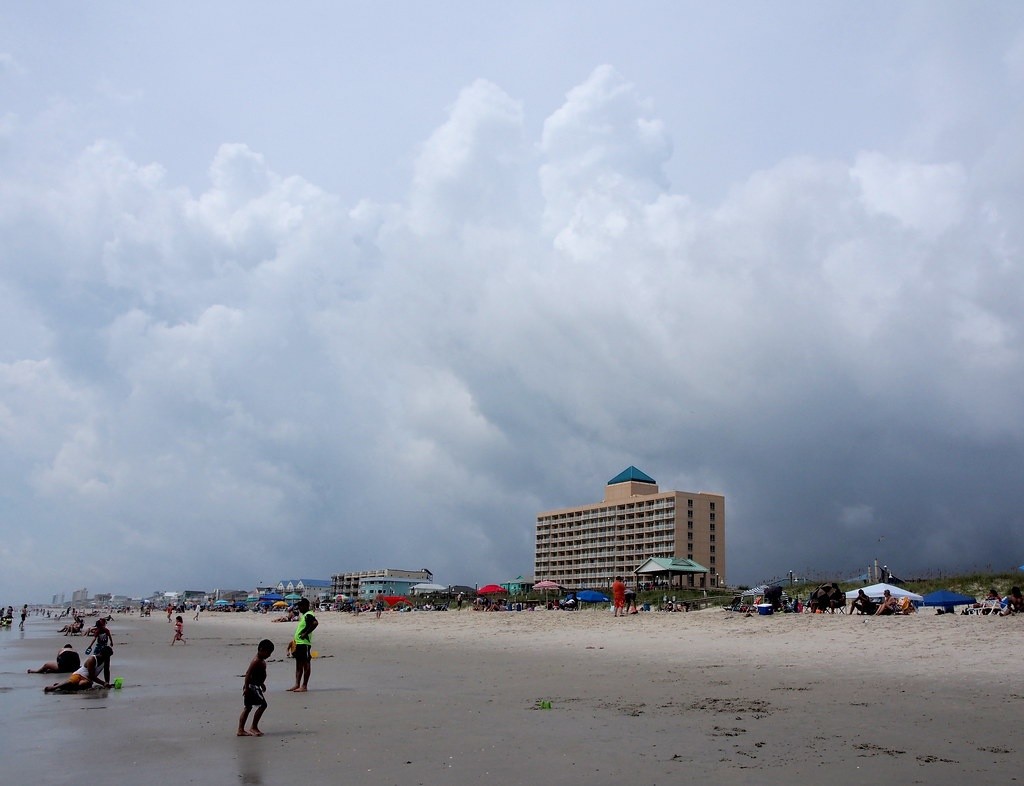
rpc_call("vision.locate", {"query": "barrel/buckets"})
[114,678,124,689]
[312,651,319,657]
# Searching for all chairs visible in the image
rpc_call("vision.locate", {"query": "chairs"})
[837,592,847,614]
[857,597,912,615]
[967,596,1008,615]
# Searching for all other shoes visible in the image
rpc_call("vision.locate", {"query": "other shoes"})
[620,615,624,617]
[615,615,617,617]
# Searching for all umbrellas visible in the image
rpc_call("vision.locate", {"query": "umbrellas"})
[333,594,348,602]
[532,581,558,609]
[477,585,506,606]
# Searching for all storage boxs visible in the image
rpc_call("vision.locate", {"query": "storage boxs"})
[757,604,773,615]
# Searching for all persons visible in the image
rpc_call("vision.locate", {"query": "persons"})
[171,615,187,646]
[0,582,1024,699]
[237,640,275,737]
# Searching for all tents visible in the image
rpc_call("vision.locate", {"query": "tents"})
[259,594,286,601]
[567,590,610,603]
[214,599,233,606]
[742,585,788,597]
[285,593,302,600]
[235,601,248,606]
[918,590,977,605]
[845,583,923,601]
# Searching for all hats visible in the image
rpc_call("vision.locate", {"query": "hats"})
[460,592,465,595]
[293,598,309,606]
[668,601,672,603]
[93,618,107,627]
